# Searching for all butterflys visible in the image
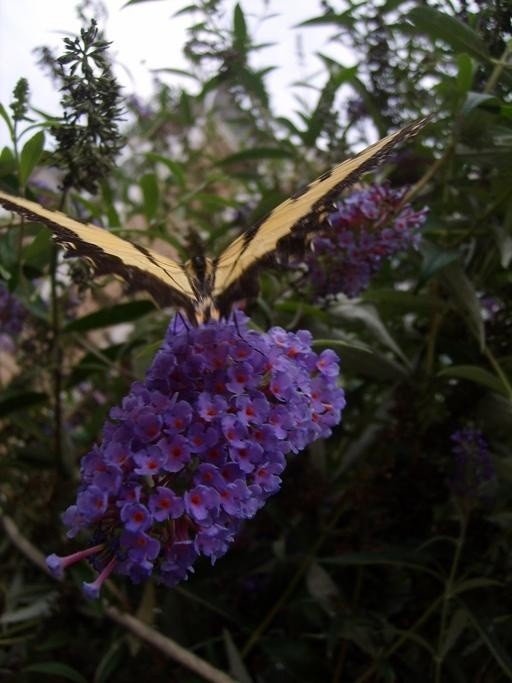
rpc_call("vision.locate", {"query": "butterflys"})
[0,111,439,359]
